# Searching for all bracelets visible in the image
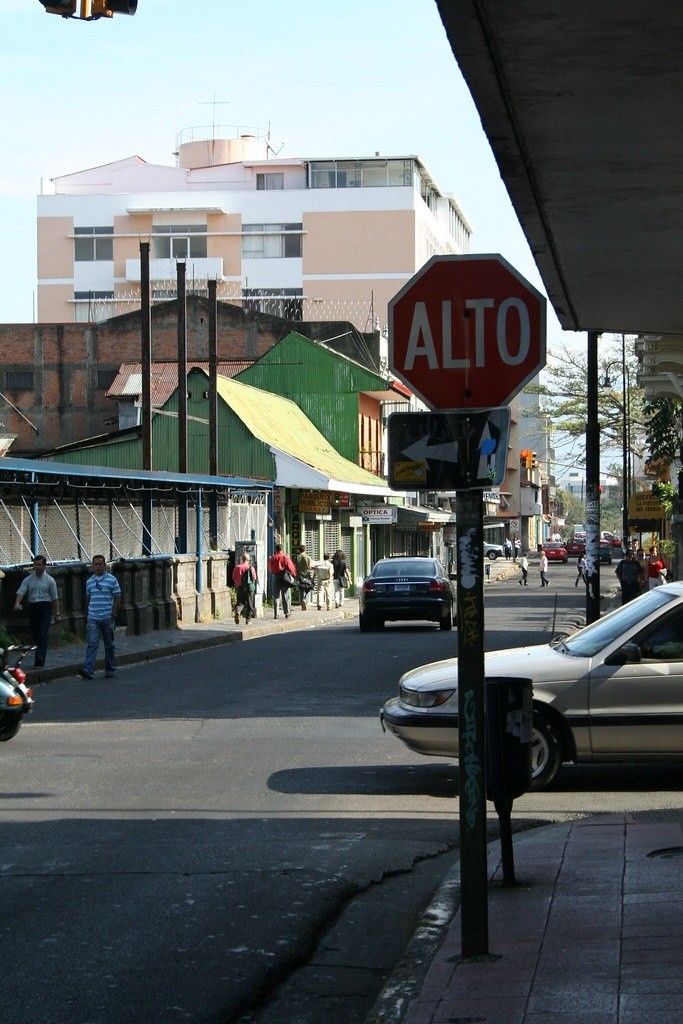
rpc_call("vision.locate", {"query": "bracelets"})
[56,613,60,616]
[651,646,653,652]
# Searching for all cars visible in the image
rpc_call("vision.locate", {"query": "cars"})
[566,538,585,555]
[359,555,456,632]
[380,579,683,793]
[541,543,568,563]
[600,531,621,548]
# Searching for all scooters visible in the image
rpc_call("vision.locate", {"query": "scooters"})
[0,642,38,741]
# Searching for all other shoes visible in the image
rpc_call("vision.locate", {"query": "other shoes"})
[274,615,279,619]
[286,613,291,618]
[519,581,522,585]
[302,600,306,610]
[547,582,550,585]
[78,669,94,680]
[317,606,321,610]
[339,576,348,588]
[105,671,114,678]
[327,609,330,611]
[540,585,546,587]
[247,621,251,625]
[524,583,528,586]
[299,576,315,590]
[235,612,240,624]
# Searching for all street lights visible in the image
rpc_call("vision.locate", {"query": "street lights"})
[603,361,632,497]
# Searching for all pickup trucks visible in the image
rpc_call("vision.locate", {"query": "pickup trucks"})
[483,541,503,560]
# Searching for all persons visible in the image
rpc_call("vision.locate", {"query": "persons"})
[515,536,521,558]
[268,544,297,619]
[518,553,529,586]
[644,636,683,659]
[330,550,352,607]
[551,532,561,542]
[615,538,670,608]
[538,551,550,587]
[79,555,121,680]
[575,553,587,586]
[14,555,61,667]
[293,546,312,610]
[232,554,256,624]
[502,538,512,560]
[314,553,334,610]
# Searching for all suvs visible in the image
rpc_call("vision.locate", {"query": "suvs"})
[599,540,612,564]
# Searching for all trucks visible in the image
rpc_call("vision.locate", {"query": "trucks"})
[574,525,586,544]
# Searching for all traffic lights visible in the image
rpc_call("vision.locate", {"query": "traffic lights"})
[521,449,527,457]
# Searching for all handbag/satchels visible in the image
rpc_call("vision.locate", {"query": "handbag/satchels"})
[242,566,254,594]
[278,570,296,587]
